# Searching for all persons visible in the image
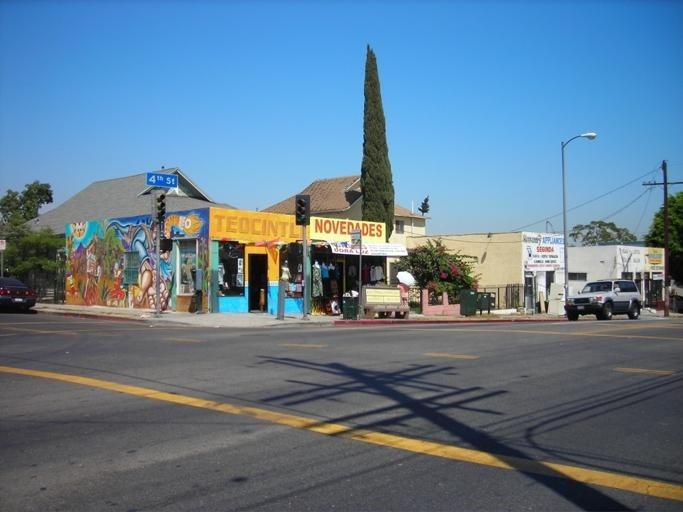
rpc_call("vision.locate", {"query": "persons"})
[311,261,324,298]
[279,259,292,281]
[318,262,329,280]
[327,263,337,282]
[396,282,409,307]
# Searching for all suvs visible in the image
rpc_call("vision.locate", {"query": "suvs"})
[563,279,644,321]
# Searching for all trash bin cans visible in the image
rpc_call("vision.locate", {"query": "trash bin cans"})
[342,297,359,320]
[537,302,547,313]
[460,290,495,317]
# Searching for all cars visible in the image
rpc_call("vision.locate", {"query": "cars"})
[0,276,37,312]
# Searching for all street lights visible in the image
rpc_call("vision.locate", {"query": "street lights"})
[560,133,598,319]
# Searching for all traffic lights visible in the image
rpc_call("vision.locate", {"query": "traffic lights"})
[295,194,310,226]
[160,238,173,253]
[152,190,167,223]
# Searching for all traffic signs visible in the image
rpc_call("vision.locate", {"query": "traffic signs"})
[146,171,179,187]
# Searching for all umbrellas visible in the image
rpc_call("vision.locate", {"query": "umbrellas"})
[396,271,415,287]
